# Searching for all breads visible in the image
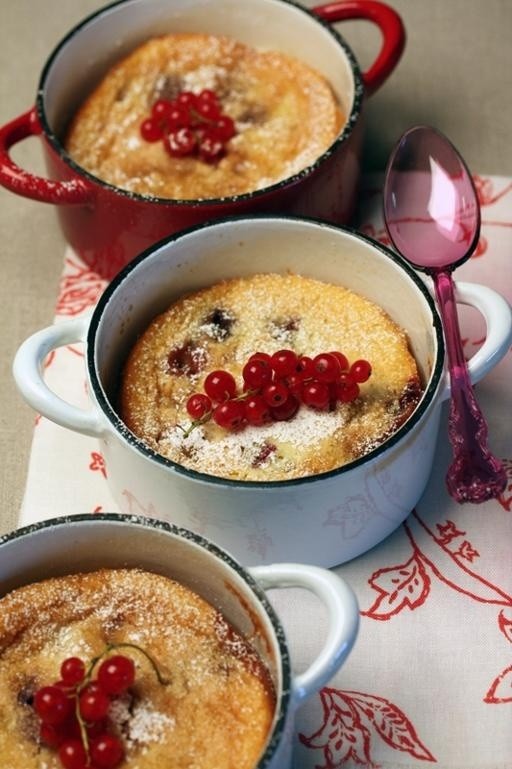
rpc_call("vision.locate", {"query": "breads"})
[66,31,344,200]
[122,271,423,484]
[0,567,275,769]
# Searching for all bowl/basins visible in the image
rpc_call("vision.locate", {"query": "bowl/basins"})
[12,214,512,568]
[0,0,407,283]
[0,513,360,769]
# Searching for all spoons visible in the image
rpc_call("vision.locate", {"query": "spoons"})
[383,124,508,505]
